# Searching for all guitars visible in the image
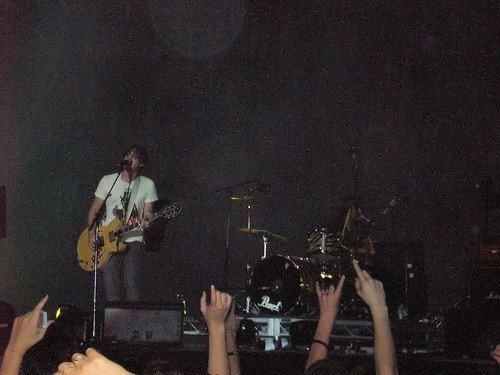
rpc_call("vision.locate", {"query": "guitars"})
[76,201,183,272]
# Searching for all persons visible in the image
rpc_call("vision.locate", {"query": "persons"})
[200,285,241,375]
[319,199,393,296]
[87,145,158,301]
[305,260,397,375]
[0,294,185,375]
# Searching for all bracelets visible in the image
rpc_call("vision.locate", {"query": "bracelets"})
[311,340,329,350]
[7,349,24,355]
[228,351,240,359]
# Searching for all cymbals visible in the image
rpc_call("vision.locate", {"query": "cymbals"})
[236,227,290,243]
[330,191,385,207]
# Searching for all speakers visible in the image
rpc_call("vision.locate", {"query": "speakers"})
[467,266,500,360]
[337,239,425,321]
[102,304,185,347]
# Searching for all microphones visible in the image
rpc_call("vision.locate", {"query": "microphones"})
[119,160,132,165]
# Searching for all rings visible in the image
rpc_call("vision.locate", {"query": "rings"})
[72,355,83,363]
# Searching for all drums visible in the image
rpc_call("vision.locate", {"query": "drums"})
[245,252,321,317]
[305,225,345,260]
